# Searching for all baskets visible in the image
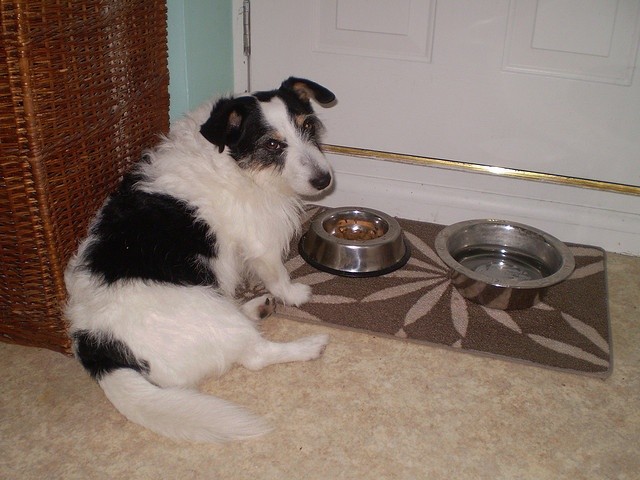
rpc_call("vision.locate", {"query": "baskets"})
[0,0,170,359]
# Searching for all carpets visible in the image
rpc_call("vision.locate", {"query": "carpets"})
[236,203,614,380]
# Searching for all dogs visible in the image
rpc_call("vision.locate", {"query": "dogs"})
[57,73,336,447]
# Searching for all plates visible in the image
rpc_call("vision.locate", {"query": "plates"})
[296,205,412,277]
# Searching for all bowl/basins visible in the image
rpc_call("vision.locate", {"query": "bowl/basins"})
[432,217,577,310]
